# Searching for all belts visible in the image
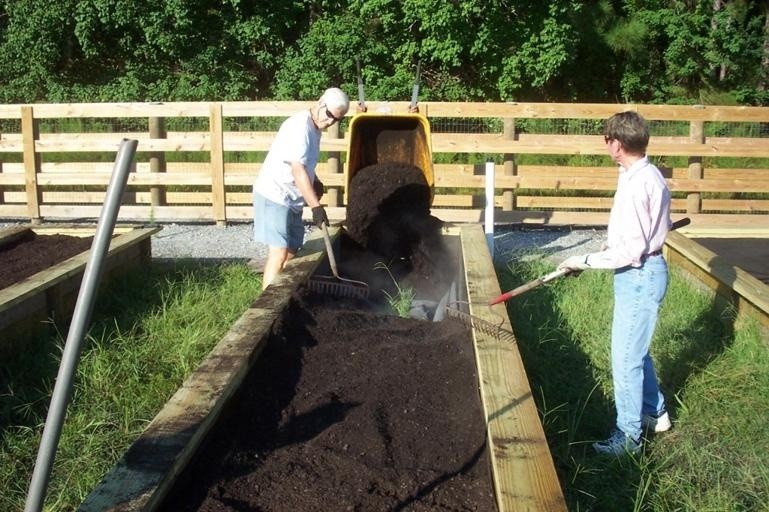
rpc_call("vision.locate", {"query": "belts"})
[643,249,663,257]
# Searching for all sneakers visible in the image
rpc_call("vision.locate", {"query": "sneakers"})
[640,412,672,434]
[590,426,643,457]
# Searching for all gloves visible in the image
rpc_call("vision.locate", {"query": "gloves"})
[559,254,589,276]
[313,182,323,200]
[311,206,330,230]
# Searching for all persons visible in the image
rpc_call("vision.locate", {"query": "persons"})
[355,101,421,116]
[251,87,348,294]
[558,112,671,455]
